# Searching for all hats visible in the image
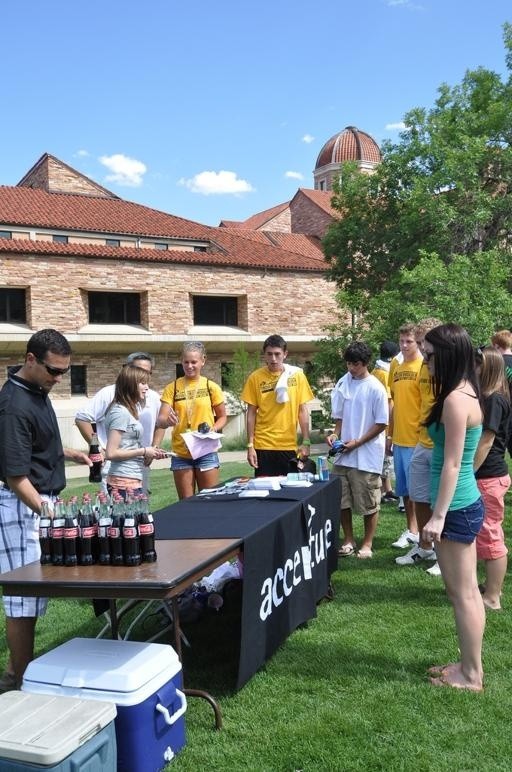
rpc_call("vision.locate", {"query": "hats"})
[122,352,153,369]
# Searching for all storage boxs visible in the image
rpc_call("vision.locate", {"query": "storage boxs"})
[0,637,188,772]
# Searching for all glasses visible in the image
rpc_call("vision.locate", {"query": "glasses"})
[423,351,435,361]
[35,358,69,377]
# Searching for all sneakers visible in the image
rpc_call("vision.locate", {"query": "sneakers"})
[426,561,442,576]
[381,497,393,502]
[395,544,439,564]
[398,496,405,512]
[385,491,399,499]
[392,530,420,548]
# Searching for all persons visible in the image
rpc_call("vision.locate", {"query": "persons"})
[154,339,229,501]
[475,327,511,610]
[0,327,72,689]
[422,322,486,693]
[74,351,168,489]
[239,333,315,477]
[370,318,441,577]
[325,340,389,560]
[102,366,170,515]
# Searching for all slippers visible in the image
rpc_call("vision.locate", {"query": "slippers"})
[428,664,452,674]
[337,540,358,556]
[358,545,372,558]
[431,676,483,690]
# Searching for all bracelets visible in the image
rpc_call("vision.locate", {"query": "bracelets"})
[246,442,254,449]
[302,438,311,447]
[330,433,339,441]
[142,446,146,457]
[354,439,357,448]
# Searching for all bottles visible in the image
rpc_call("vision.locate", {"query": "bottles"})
[318,457,329,482]
[38,486,159,565]
[88,433,101,483]
